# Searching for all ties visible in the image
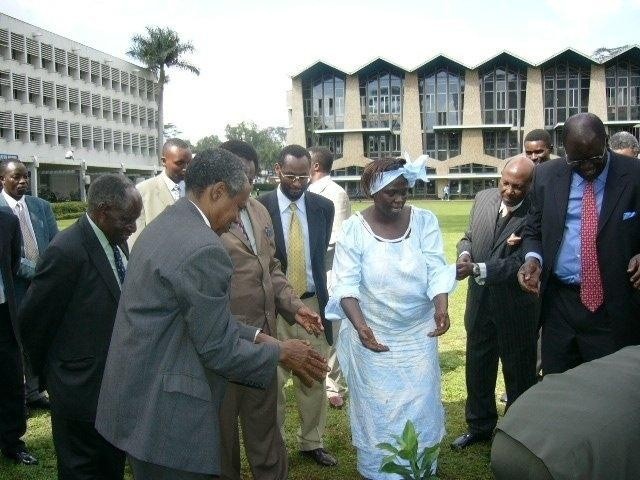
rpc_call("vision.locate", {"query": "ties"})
[109,240,126,285]
[287,203,307,298]
[236,219,255,254]
[16,202,39,264]
[173,184,181,202]
[580,180,604,313]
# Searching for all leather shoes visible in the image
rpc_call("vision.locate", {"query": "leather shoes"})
[1,446,39,465]
[450,431,493,451]
[328,396,344,409]
[26,396,51,409]
[500,393,508,402]
[300,448,335,466]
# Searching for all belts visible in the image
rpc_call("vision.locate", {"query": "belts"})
[300,292,315,299]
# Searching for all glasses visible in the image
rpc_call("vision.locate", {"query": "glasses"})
[279,168,312,186]
[563,135,609,168]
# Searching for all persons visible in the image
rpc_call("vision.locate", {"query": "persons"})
[257,190,260,198]
[124,138,193,254]
[19,172,143,480]
[325,155,458,480]
[608,130,639,162]
[516,112,640,379]
[94,149,331,480]
[308,143,351,412]
[451,157,535,451]
[254,145,339,466]
[0,156,60,407]
[353,187,362,203]
[219,139,326,480]
[441,183,449,201]
[524,129,554,167]
[489,343,639,479]
[0,205,39,468]
